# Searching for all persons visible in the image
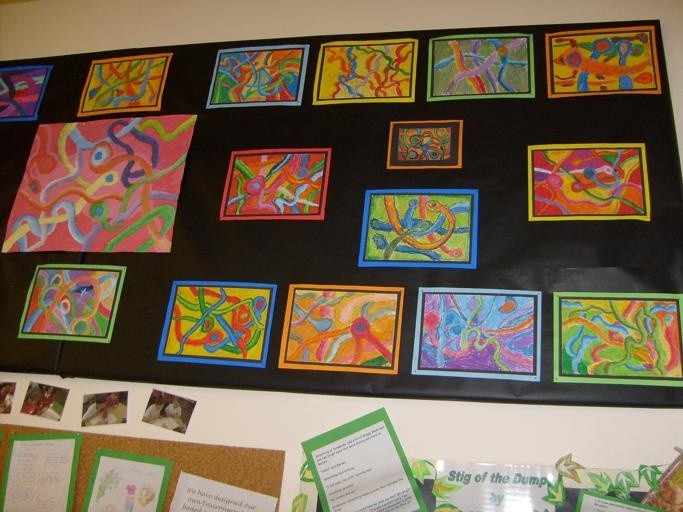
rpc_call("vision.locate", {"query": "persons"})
[31,400,43,416]
[143,397,165,420]
[37,386,56,416]
[0,384,11,404]
[102,393,120,418]
[82,399,103,423]
[165,398,181,417]
